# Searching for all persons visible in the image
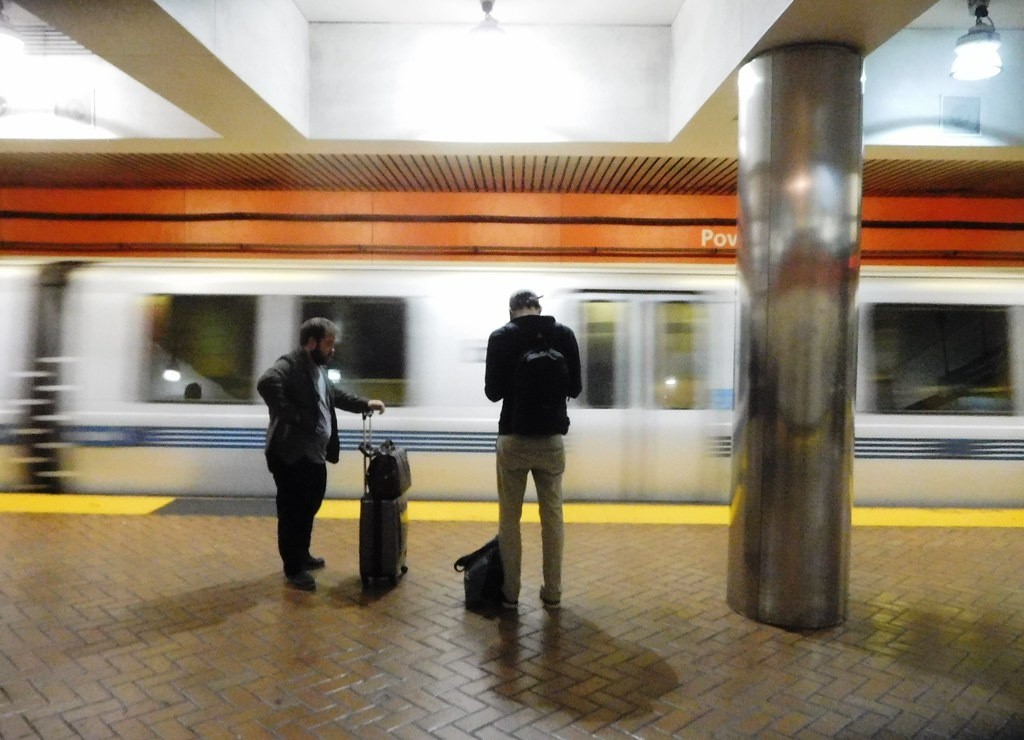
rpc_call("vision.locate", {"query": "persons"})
[257,318,385,590]
[485,291,583,608]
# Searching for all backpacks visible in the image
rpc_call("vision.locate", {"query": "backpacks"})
[504,322,569,435]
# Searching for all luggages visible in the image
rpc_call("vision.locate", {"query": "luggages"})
[359,409,408,588]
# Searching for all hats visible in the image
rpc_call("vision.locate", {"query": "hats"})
[509,290,544,321]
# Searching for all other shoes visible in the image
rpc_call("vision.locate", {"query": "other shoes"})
[300,553,325,570]
[284,566,316,589]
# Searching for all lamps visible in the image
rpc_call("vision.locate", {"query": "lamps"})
[468,0,506,36]
[950,0,1003,81]
[162,344,181,381]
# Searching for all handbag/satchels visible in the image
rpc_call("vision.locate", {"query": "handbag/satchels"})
[454,535,503,608]
[358,440,411,500]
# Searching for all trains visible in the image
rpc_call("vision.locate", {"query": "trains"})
[0,180,1023,513]
